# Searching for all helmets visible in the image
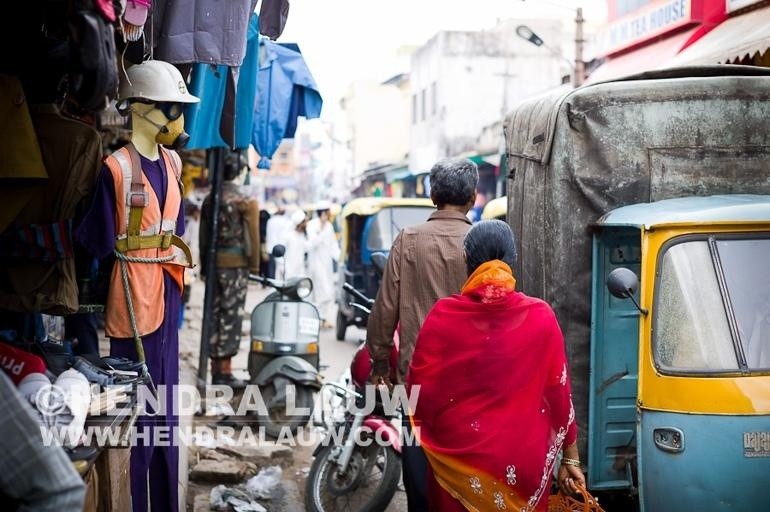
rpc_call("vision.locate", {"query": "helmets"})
[119,59,201,108]
[222,153,250,181]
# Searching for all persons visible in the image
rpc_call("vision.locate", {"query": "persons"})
[201,157,261,391]
[364,158,480,511]
[91,61,200,511]
[260,189,344,337]
[406,218,588,511]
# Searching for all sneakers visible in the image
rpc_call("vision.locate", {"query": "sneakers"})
[216,373,248,390]
[48,367,91,448]
[19,372,52,424]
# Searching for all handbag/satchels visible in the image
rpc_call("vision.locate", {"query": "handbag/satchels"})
[546,475,609,512]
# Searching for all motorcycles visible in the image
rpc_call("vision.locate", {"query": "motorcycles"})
[333,197,455,343]
[242,244,324,440]
[307,282,407,512]
[497,63,768,512]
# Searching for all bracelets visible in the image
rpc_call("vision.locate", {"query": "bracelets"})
[560,458,583,467]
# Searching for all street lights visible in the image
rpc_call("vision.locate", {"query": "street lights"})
[513,24,585,87]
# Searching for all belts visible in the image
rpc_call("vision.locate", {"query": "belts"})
[115,228,193,267]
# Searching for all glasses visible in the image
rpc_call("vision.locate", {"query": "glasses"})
[139,98,186,122]
